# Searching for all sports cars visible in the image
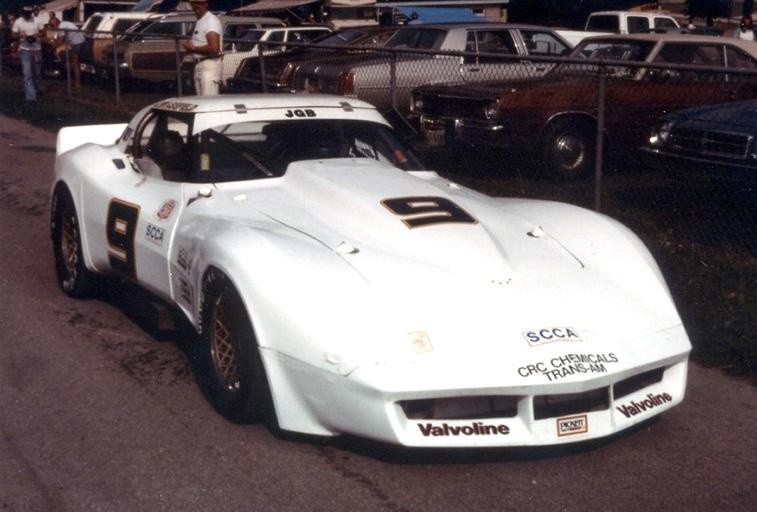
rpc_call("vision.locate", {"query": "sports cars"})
[48,90,695,448]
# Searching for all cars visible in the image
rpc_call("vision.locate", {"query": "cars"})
[291,23,590,102]
[408,30,757,177]
[0,11,410,107]
[632,97,757,192]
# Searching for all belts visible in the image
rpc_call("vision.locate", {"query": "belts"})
[195,54,222,62]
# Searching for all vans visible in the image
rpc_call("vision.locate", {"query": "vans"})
[586,11,683,37]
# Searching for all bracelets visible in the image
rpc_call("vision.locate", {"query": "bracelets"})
[190,45,196,53]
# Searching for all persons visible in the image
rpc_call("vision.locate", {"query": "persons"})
[8,5,47,106]
[48,10,62,31]
[735,12,756,42]
[181,0,224,97]
[50,18,87,91]
[31,5,50,33]
